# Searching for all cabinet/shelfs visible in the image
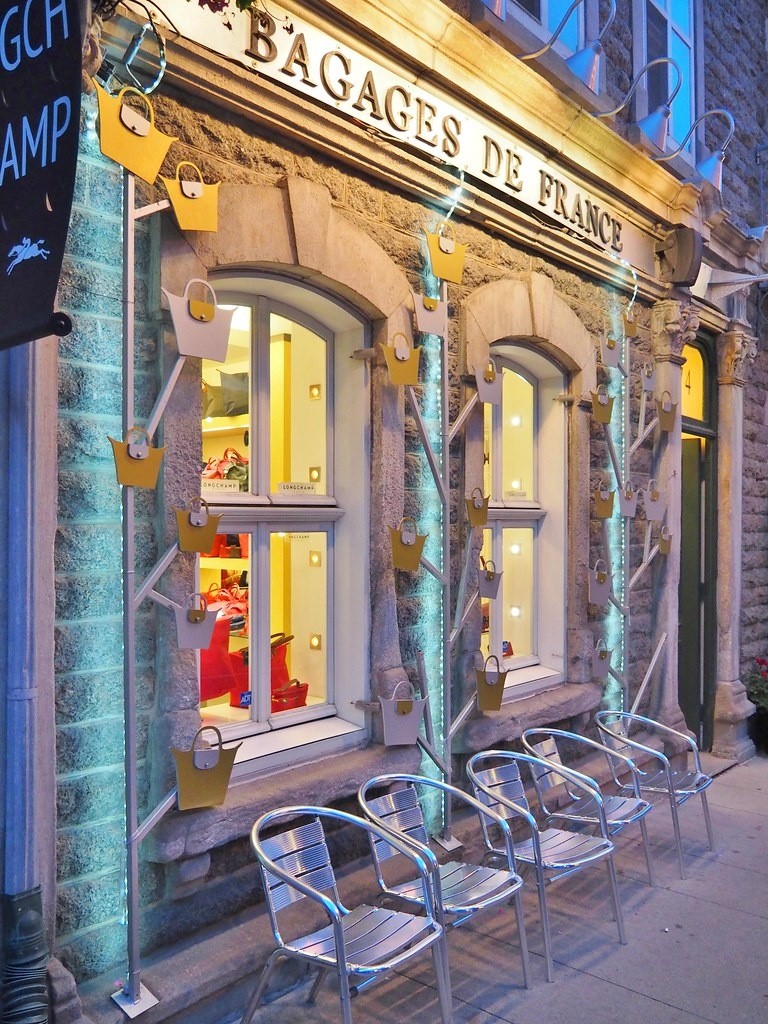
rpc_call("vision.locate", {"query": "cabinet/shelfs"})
[199,332,291,708]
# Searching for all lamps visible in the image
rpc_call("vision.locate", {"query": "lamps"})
[592,56,682,154]
[481,0,506,22]
[515,0,617,96]
[649,107,736,192]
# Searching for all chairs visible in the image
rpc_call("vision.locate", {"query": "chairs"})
[469,750,628,984]
[237,804,452,1024]
[520,727,657,921]
[359,773,533,1024]
[594,709,715,880]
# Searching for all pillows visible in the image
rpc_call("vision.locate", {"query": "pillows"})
[199,378,227,419]
[215,367,248,417]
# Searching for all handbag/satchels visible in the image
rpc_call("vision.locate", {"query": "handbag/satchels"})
[215,368,249,416]
[228,633,294,709]
[200,533,249,558]
[200,449,249,492]
[487,641,513,657]
[201,378,226,418]
[271,679,308,713]
[199,582,249,701]
[481,616,489,632]
